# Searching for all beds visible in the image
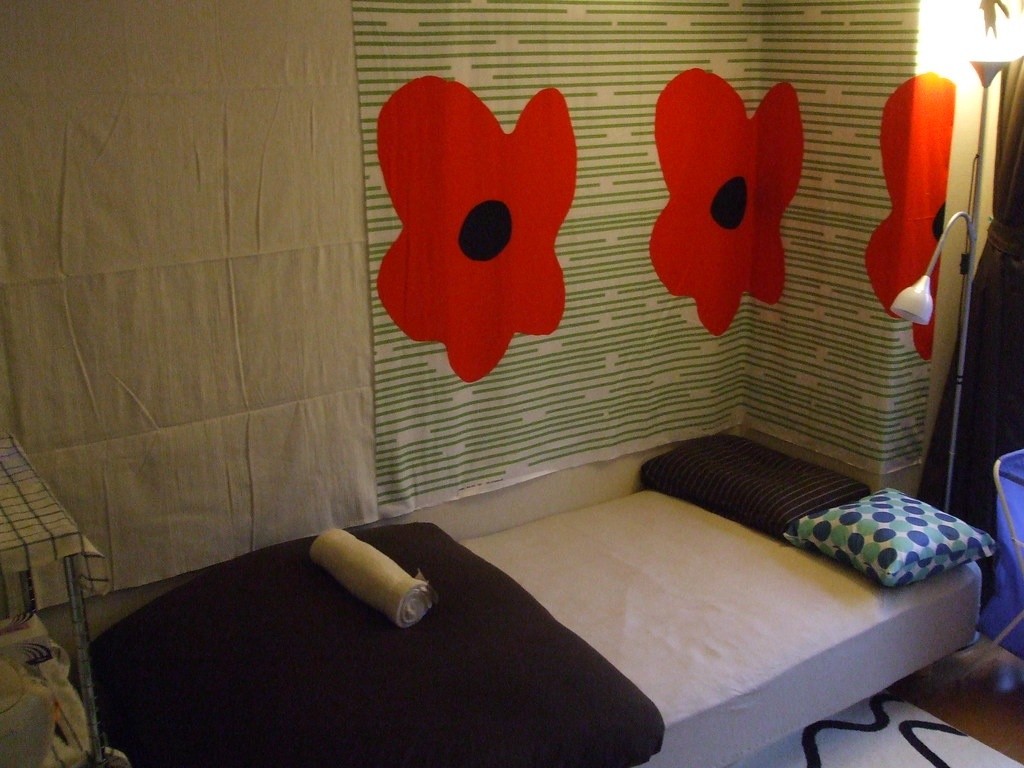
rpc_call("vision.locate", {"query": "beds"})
[88,492,983,768]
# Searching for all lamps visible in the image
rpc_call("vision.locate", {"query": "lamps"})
[890,32,1024,513]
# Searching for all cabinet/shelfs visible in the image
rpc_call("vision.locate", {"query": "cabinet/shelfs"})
[0,429,132,768]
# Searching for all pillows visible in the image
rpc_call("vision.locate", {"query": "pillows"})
[783,486,1000,588]
[640,433,871,541]
